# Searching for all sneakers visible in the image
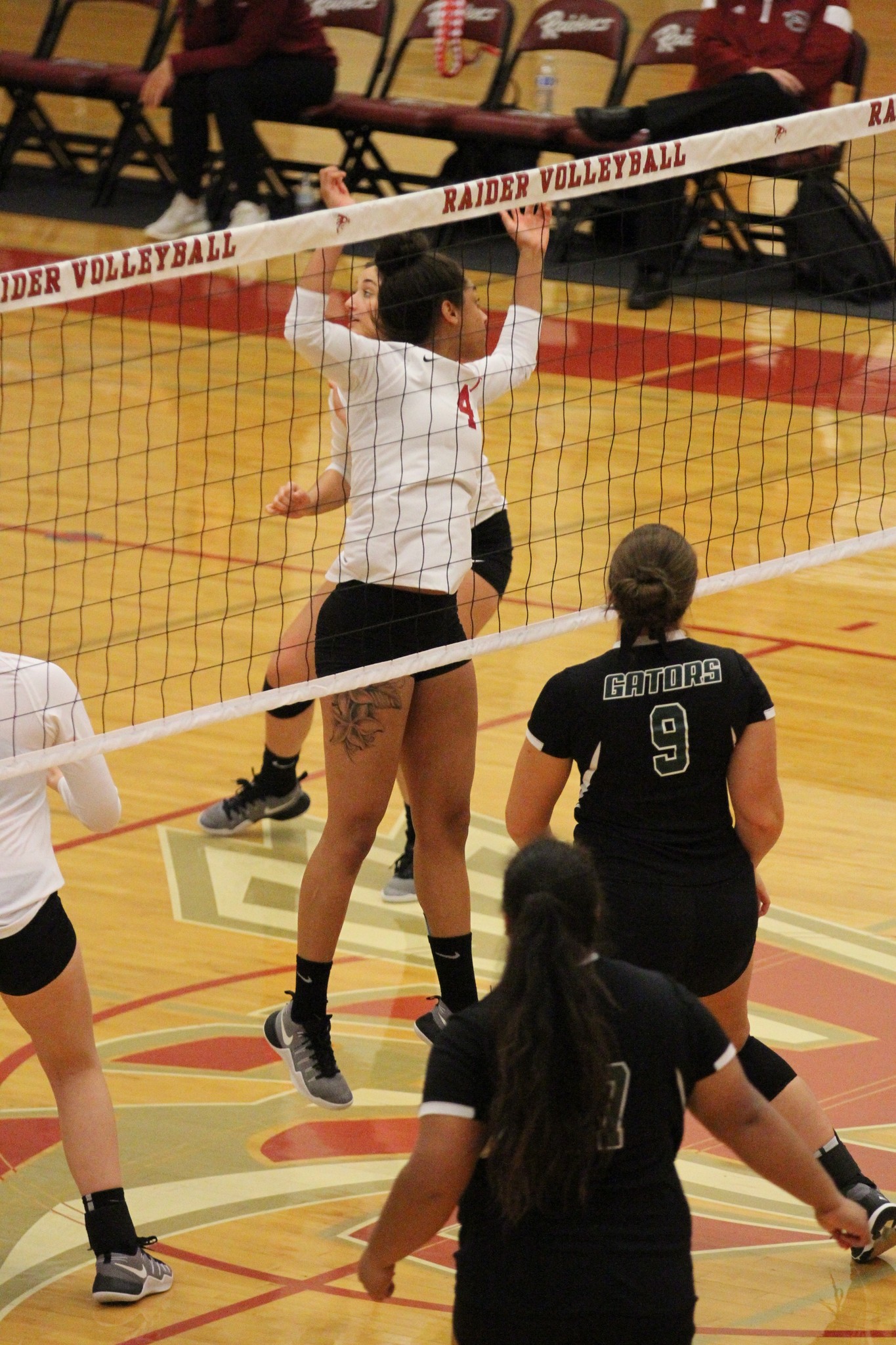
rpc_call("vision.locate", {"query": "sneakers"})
[412,995,453,1047]
[197,766,311,836]
[380,828,418,903]
[224,201,269,229]
[845,1182,896,1263]
[263,990,354,1110]
[144,192,211,240]
[92,1236,174,1303]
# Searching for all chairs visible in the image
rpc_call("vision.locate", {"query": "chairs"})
[0,0,870,284]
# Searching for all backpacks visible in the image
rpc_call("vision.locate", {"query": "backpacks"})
[785,176,896,304]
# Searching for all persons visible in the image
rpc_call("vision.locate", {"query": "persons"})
[0,652,178,1308]
[575,0,854,310]
[263,165,554,1111]
[355,837,871,1345]
[138,0,338,241]
[506,521,896,1263]
[198,233,514,904]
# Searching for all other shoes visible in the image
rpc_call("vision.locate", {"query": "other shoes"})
[626,270,672,310]
[573,104,635,143]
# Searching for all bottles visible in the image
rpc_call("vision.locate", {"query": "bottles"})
[295,175,314,215]
[534,56,554,113]
